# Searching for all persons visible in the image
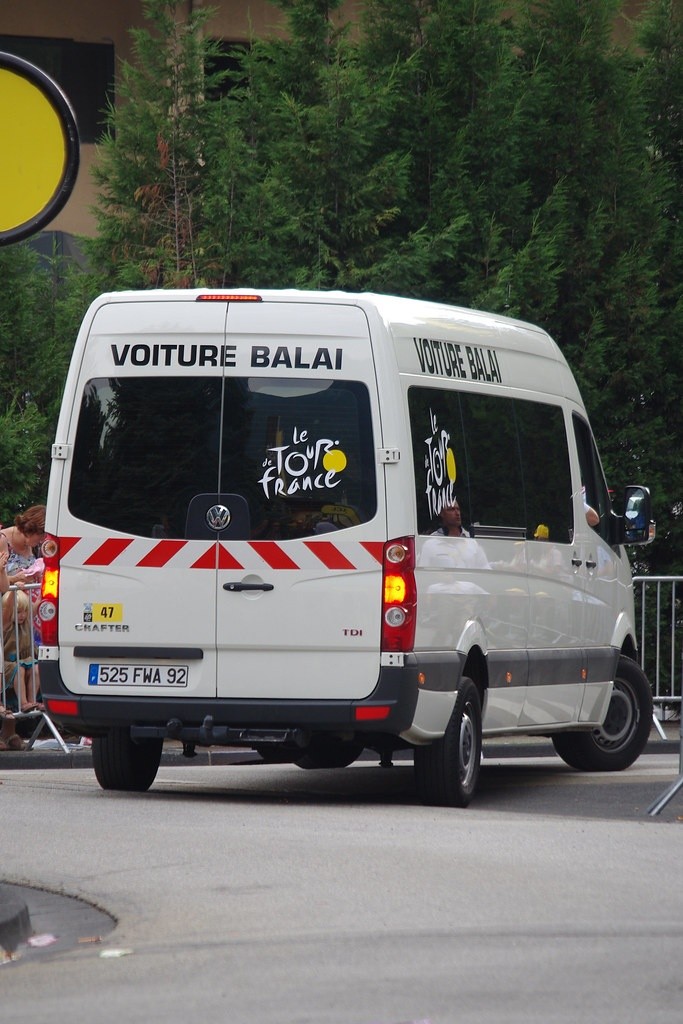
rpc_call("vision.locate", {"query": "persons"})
[510,524,564,573]
[0,506,46,587]
[418,498,490,569]
[584,502,600,526]
[2,581,45,713]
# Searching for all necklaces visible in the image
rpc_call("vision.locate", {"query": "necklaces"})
[12,525,26,552]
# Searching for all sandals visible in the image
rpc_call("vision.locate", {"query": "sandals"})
[0,734,33,750]
[0,704,15,721]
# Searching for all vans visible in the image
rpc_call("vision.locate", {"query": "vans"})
[35,285,656,809]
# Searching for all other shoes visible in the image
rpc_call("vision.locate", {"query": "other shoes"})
[39,703,45,711]
[21,702,38,713]
